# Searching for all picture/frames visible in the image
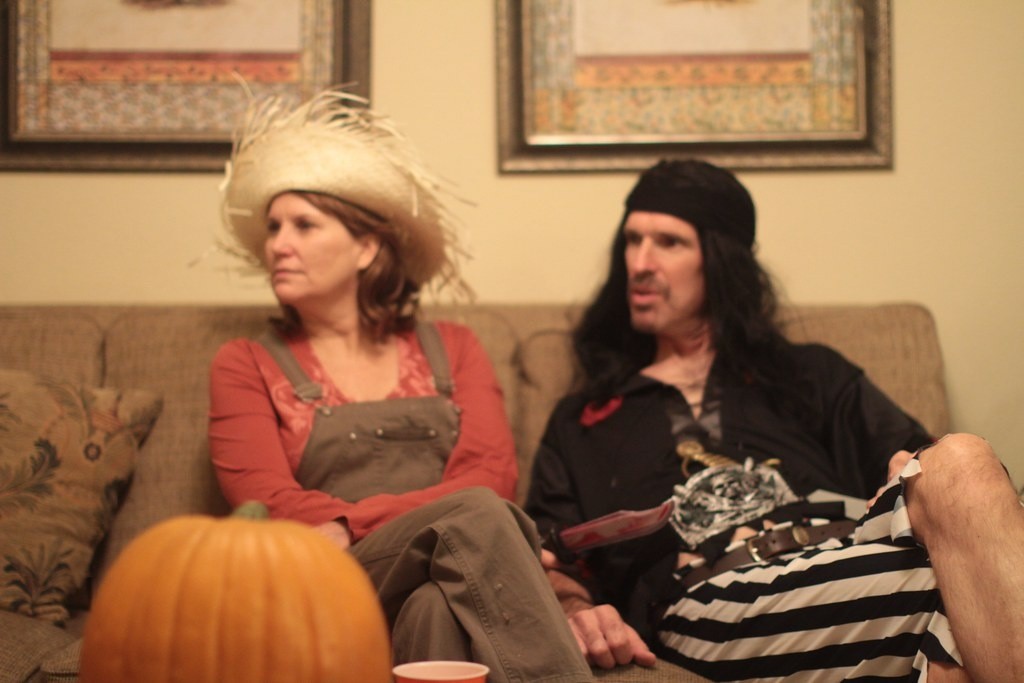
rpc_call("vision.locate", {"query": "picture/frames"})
[495,0,897,174]
[0,0,371,170]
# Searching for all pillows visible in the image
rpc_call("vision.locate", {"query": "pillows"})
[0,367,164,621]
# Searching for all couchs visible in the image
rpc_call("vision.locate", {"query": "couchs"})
[0,299,952,683]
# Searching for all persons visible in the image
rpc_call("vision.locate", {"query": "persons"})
[523,160,1024,683]
[205,68,600,683]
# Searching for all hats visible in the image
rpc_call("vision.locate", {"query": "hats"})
[216,71,484,304]
[627,160,757,248]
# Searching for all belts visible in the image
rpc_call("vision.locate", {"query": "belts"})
[683,520,857,587]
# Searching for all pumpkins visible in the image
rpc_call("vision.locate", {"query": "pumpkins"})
[79,514,391,683]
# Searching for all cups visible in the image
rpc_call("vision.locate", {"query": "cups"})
[392,661,490,683]
